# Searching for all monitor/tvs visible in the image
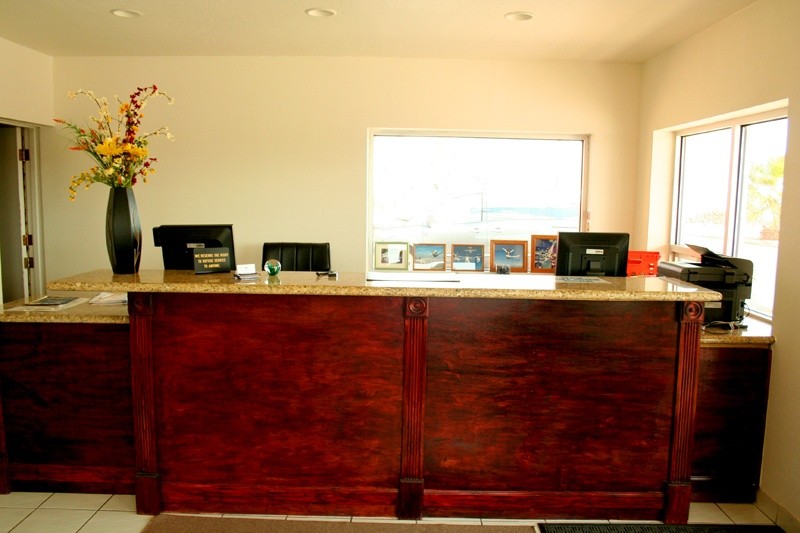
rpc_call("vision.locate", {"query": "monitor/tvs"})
[556,232,629,277]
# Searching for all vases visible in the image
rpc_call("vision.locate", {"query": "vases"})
[106,186,143,274]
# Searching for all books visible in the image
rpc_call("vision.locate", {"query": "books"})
[89,291,127,305]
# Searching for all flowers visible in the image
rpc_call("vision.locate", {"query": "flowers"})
[53,84,175,197]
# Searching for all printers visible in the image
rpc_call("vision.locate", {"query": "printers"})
[656,243,753,323]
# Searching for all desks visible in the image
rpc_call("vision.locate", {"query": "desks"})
[691,326,775,509]
[47,272,722,525]
[0,294,137,494]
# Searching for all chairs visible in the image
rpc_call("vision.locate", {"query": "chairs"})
[262,242,330,271]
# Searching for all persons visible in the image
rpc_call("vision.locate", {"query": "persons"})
[550,254,556,268]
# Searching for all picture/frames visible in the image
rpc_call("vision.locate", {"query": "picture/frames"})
[451,244,484,271]
[531,235,559,274]
[412,243,446,271]
[490,240,528,272]
[375,243,408,269]
